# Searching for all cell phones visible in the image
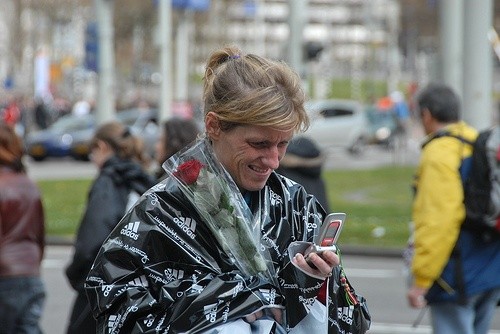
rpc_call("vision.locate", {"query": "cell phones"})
[304,213,347,259]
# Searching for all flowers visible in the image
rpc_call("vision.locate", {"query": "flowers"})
[163,137,281,289]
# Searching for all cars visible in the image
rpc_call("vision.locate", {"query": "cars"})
[69,108,159,160]
[290,98,369,155]
[21,114,95,161]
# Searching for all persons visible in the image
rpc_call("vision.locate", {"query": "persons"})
[386,83,416,139]
[66,47,374,333]
[406,74,499,332]
[67,112,164,332]
[0,109,48,334]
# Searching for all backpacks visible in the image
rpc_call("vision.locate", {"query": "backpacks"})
[420,128,500,244]
